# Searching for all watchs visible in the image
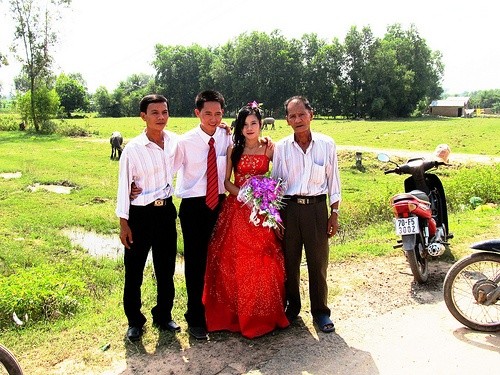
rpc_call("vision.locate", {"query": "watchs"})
[332,209,339,215]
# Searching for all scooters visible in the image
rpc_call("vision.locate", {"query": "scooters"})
[442,238,500,332]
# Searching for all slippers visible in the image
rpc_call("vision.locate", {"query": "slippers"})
[313,314,335,332]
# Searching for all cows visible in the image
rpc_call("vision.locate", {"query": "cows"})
[261,117,275,130]
[109,130,124,161]
[461,108,477,119]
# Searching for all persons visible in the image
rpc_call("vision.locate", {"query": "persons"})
[271,97,341,333]
[115,94,231,337]
[129,90,273,338]
[202,102,289,338]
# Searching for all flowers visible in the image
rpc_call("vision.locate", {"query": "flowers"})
[234,174,289,232]
[248,101,264,114]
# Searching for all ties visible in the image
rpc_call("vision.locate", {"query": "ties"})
[206,137,218,210]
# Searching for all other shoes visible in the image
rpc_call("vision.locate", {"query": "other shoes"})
[124,326,144,340]
[186,324,207,339]
[153,319,180,331]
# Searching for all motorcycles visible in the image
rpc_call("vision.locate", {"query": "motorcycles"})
[378,152,454,285]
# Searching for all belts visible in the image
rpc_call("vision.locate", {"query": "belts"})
[147,196,174,206]
[282,195,326,204]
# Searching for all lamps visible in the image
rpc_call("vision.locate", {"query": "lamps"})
[355,152,363,161]
